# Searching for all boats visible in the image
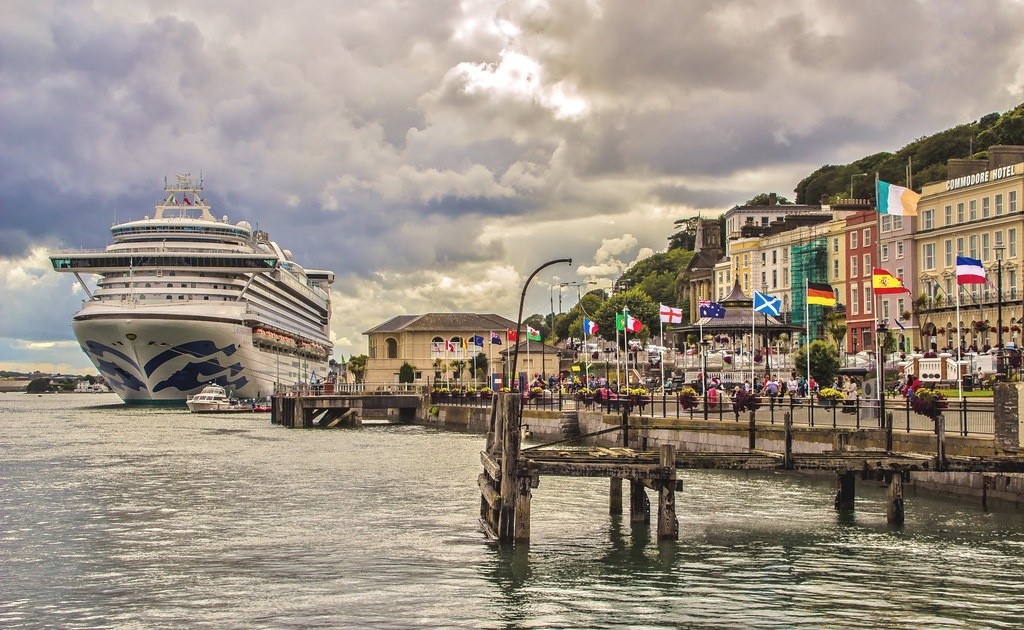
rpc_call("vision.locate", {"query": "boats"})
[73,380,108,393]
[185,378,272,414]
[253,329,326,359]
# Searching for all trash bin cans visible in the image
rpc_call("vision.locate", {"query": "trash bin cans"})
[995,373,1006,382]
[963,375,972,391]
[324,383,334,395]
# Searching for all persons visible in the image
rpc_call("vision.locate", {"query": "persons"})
[525,342,1020,417]
[293,378,324,395]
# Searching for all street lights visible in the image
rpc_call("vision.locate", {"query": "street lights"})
[500,356,506,392]
[852,330,859,367]
[433,362,439,403]
[761,282,771,380]
[875,319,889,429]
[691,267,714,302]
[960,328,967,351]
[459,360,464,406]
[555,349,564,411]
[602,286,625,302]
[561,282,597,351]
[618,279,631,294]
[550,282,576,346]
[602,347,613,415]
[699,337,710,420]
[992,241,1008,383]
[539,318,548,381]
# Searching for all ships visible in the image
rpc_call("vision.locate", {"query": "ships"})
[47,168,335,406]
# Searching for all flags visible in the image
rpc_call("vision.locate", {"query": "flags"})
[167,193,199,205]
[875,179,920,217]
[433,256,986,353]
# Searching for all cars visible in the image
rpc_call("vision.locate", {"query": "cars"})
[566,337,602,354]
[716,349,738,356]
[760,347,777,355]
[630,339,668,355]
